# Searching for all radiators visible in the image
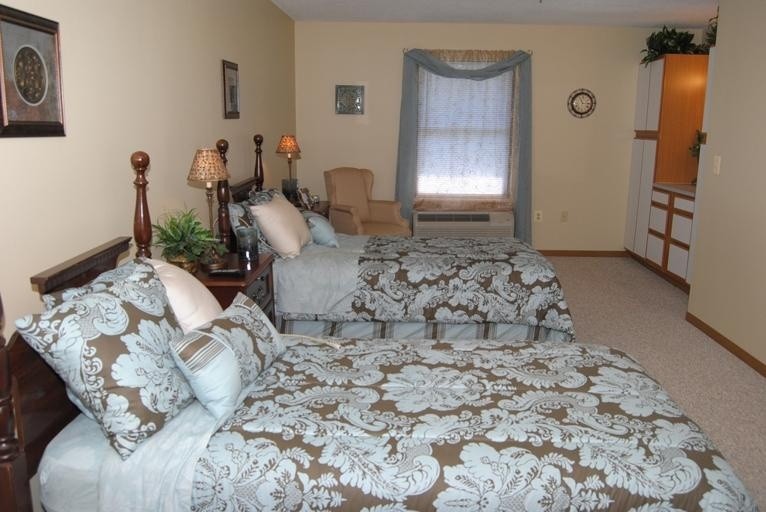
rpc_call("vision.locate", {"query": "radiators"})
[412,211,514,237]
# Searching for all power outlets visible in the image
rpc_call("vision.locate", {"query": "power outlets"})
[534,211,542,221]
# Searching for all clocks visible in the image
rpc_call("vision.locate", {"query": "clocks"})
[567,88,596,119]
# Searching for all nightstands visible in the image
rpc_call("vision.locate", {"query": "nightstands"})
[294,197,330,220]
[188,251,275,328]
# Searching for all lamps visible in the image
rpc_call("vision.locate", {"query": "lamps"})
[185,149,230,238]
[276,135,300,200]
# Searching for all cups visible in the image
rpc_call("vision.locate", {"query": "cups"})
[236,226,260,261]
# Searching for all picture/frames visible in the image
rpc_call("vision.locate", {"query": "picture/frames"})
[222,58,240,119]
[0,3,65,139]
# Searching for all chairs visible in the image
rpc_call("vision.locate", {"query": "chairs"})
[323,166,413,237]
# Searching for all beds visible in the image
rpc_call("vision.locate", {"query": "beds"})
[217,134,577,340]
[0,149,754,511]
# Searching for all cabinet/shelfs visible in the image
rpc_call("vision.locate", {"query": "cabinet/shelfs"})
[647,180,696,280]
[622,54,709,261]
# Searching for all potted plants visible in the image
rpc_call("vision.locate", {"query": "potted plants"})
[639,25,696,68]
[152,206,226,274]
